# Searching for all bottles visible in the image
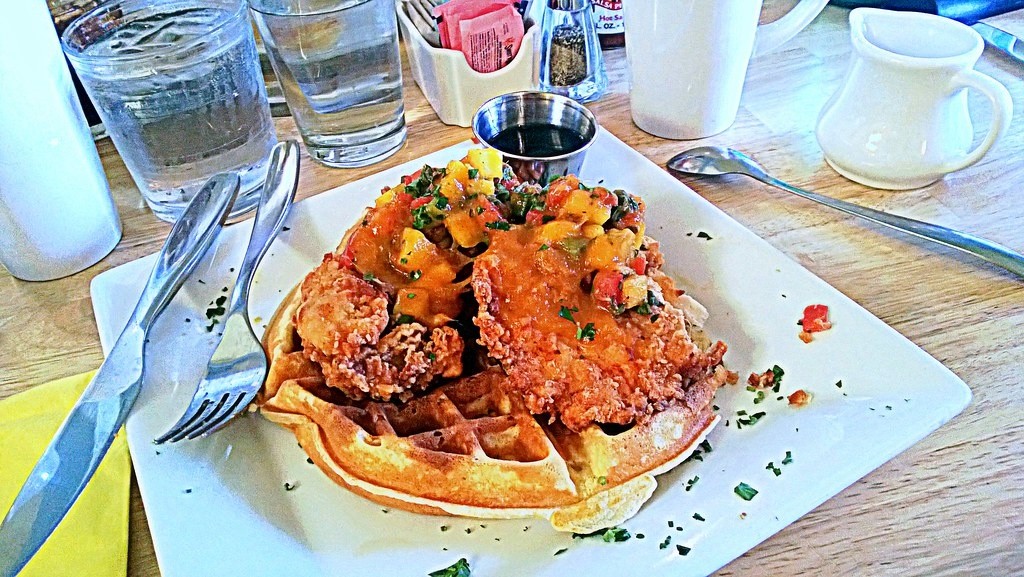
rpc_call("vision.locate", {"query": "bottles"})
[1,1,127,282]
[538,0,608,103]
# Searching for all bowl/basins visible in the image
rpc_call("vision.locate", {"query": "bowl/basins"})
[473,88,598,193]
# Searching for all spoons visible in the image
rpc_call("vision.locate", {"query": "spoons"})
[667,144,1024,280]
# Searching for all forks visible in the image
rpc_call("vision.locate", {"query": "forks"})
[150,138,302,444]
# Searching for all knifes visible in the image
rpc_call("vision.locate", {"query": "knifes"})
[2,170,244,576]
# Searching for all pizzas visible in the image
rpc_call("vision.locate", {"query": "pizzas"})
[256,148,726,535]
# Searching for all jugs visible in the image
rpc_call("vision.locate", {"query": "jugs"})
[621,0,831,140]
[816,6,1013,191]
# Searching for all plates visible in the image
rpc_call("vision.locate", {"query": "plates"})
[91,116,970,577]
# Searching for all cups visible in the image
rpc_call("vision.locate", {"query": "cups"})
[250,0,409,168]
[62,0,296,228]
